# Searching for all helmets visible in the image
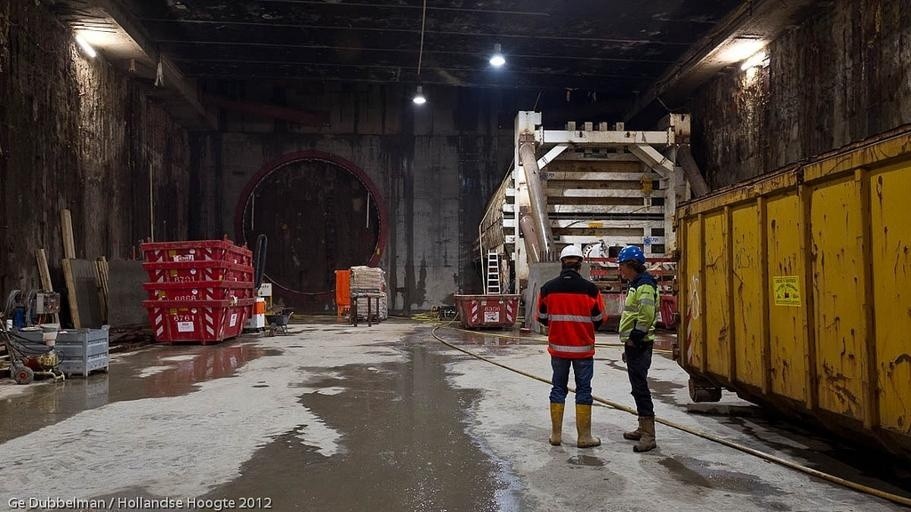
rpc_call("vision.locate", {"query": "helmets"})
[559,244,585,263]
[616,245,646,264]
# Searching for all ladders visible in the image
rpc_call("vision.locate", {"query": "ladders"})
[486,249,501,296]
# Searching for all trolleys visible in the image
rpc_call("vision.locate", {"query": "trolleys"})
[263,312,294,337]
[0,318,65,385]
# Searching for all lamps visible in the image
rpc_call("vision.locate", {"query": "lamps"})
[413,0,427,106]
[490,42,506,67]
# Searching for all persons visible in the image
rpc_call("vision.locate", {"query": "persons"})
[617,245,661,452]
[534,245,608,449]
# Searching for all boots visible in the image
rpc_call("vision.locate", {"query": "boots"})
[575,404,601,448]
[549,402,565,445]
[624,415,657,452]
